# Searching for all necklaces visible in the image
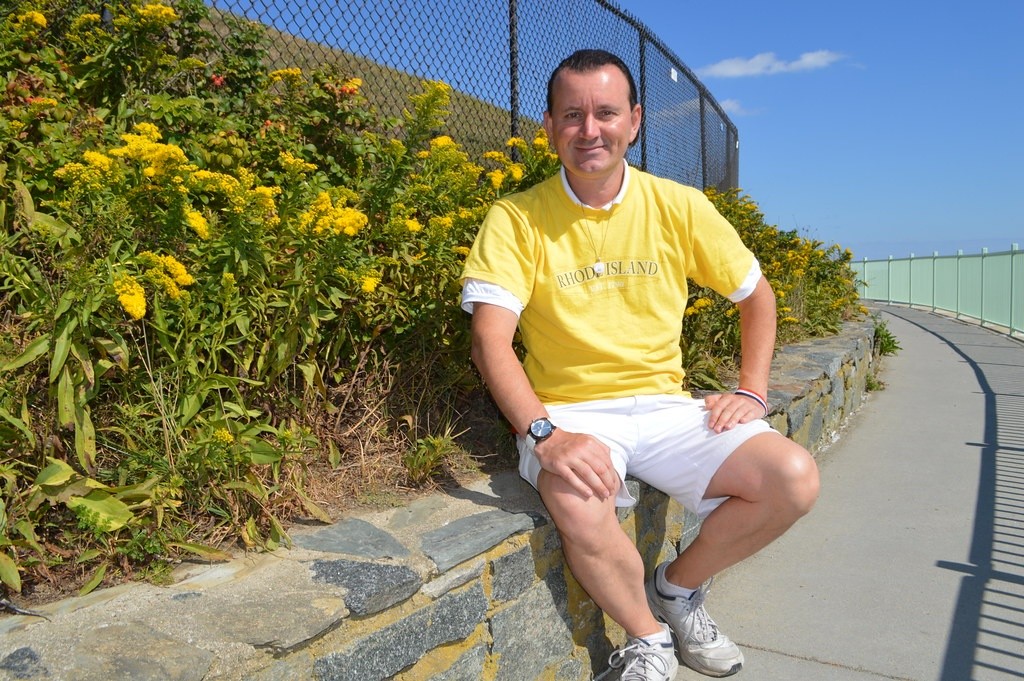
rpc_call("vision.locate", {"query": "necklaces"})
[578,196,619,274]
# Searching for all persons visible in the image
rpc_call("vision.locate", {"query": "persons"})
[456,48,822,680]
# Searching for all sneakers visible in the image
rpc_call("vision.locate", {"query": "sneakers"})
[646,561,743,679]
[623,623,679,680]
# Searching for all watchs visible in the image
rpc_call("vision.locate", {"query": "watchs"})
[525,416,557,458]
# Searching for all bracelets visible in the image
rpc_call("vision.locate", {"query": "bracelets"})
[734,387,769,421]
